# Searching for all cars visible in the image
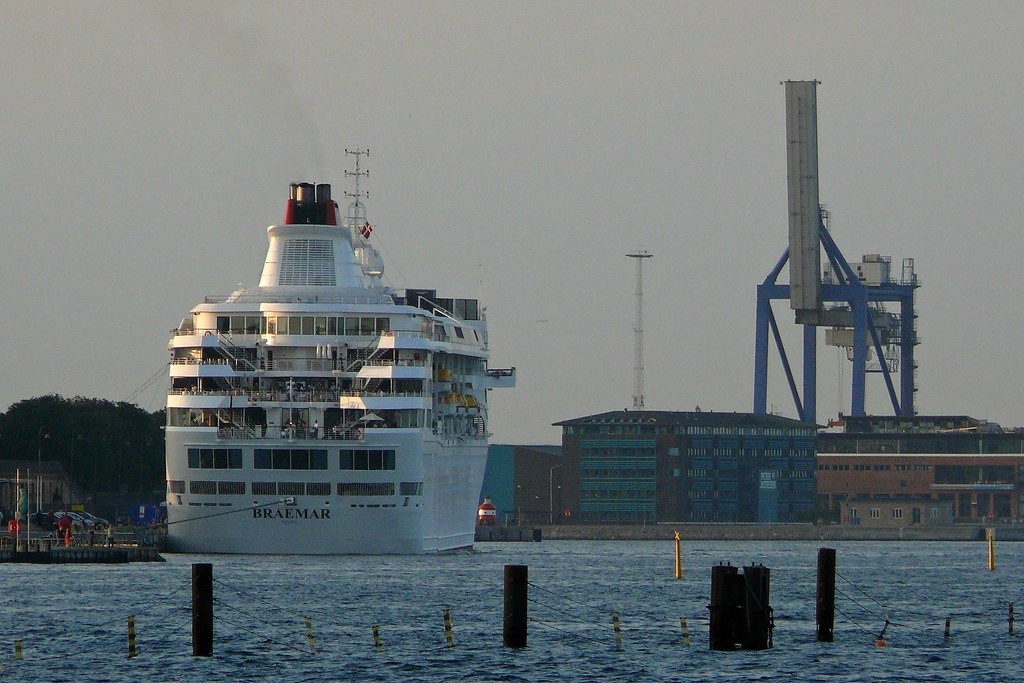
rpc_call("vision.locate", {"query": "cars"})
[32,510,109,530]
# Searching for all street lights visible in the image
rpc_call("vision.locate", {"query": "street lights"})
[549,464,565,522]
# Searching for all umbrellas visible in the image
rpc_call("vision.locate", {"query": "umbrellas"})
[359,412,385,422]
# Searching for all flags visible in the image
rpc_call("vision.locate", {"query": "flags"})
[361,221,372,239]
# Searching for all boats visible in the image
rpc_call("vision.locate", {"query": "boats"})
[163,179,490,554]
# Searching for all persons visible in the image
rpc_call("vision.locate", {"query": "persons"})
[313,419,319,439]
[88,526,95,547]
[191,384,198,394]
[287,382,338,402]
[107,524,115,547]
